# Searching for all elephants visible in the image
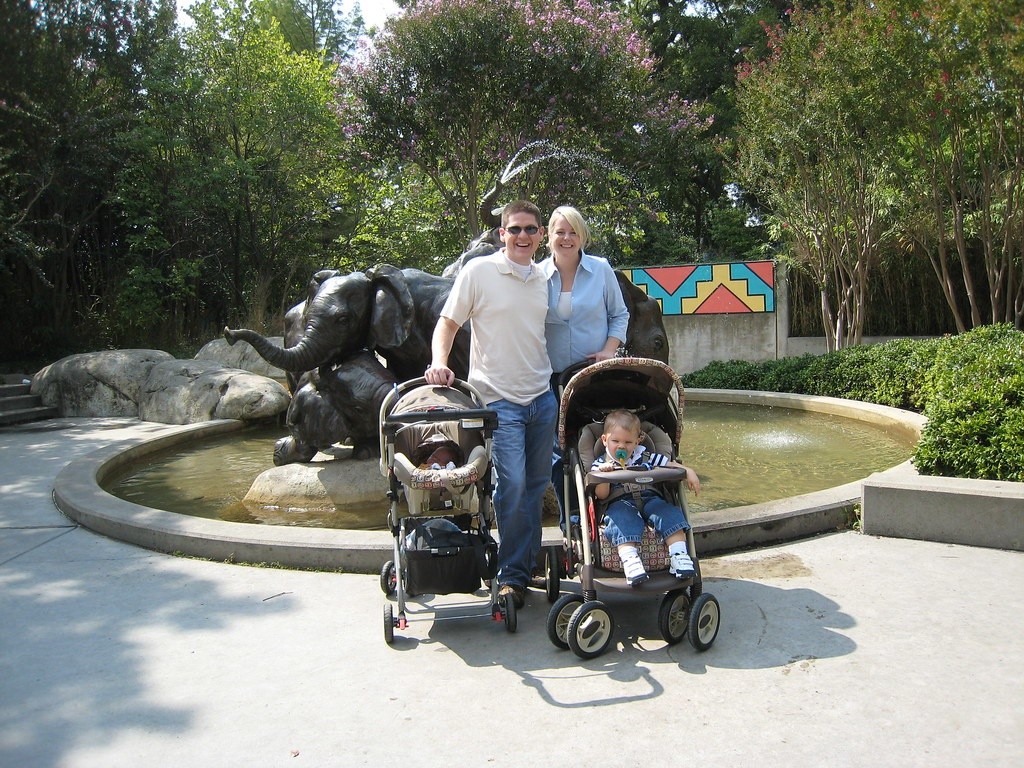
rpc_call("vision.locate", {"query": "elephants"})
[224,181,670,466]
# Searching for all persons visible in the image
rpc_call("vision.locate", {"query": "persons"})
[417,446,462,471]
[424,201,557,610]
[534,205,630,550]
[591,410,700,586]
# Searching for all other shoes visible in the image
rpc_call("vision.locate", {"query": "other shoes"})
[496,584,525,609]
[531,575,548,588]
[562,537,577,553]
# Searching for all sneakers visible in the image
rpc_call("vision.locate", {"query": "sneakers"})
[622,553,649,588]
[669,554,699,579]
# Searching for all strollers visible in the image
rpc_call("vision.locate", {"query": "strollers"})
[377,376,516,643]
[544,355,721,661]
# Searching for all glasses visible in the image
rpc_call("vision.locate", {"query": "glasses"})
[504,225,540,235]
